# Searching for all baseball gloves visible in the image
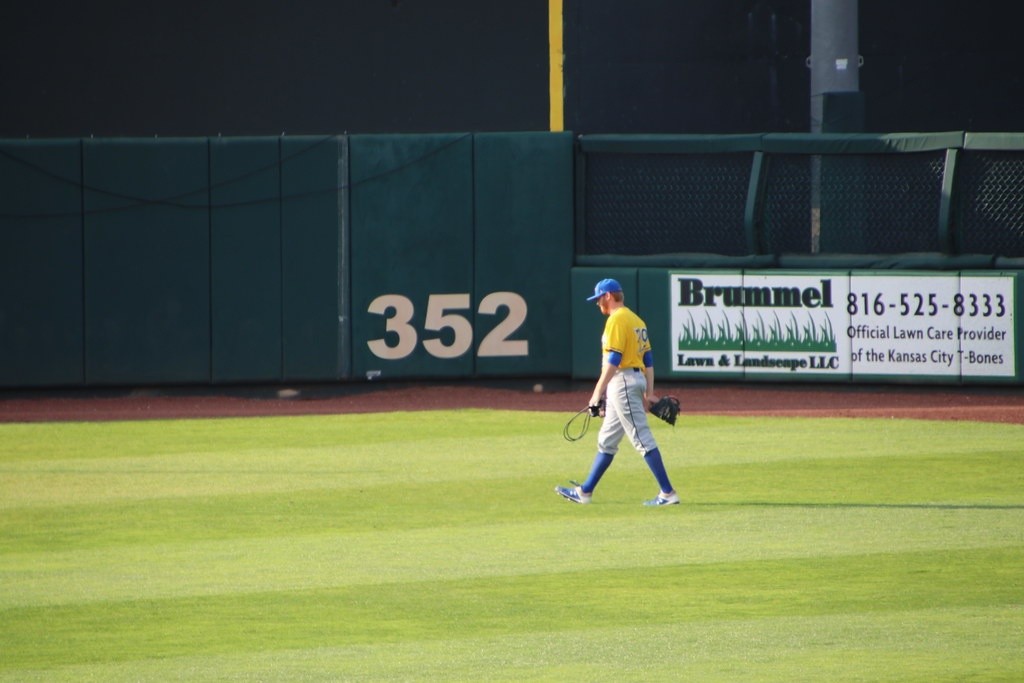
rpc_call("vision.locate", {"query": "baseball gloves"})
[647,395,680,427]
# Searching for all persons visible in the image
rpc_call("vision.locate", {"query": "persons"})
[555,279,680,506]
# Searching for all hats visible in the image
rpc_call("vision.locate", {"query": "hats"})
[586,278,623,302]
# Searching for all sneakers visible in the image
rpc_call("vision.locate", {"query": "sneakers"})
[643,488,680,507]
[554,480,592,505]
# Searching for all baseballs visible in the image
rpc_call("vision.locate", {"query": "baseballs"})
[533,383,543,393]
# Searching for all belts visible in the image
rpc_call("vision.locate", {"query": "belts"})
[633,367,644,372]
